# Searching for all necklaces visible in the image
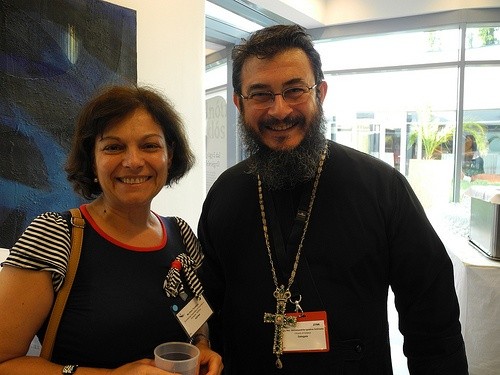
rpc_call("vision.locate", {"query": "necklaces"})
[257,139,329,369]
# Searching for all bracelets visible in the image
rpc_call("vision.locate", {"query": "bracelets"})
[188,334,210,349]
[63,363,80,375]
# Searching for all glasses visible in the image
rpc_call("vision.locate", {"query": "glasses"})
[241,84,319,109]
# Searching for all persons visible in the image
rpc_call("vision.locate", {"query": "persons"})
[0,85,225,375]
[198,26,471,375]
[462,135,483,179]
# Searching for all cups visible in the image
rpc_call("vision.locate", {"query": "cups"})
[154,342,200,375]
